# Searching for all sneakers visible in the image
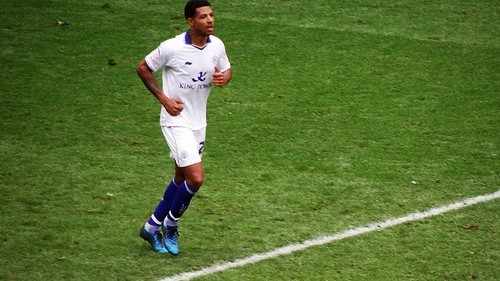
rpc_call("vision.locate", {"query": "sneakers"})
[140,223,168,255]
[160,221,180,257]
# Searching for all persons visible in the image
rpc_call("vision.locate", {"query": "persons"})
[137,0,233,255]
